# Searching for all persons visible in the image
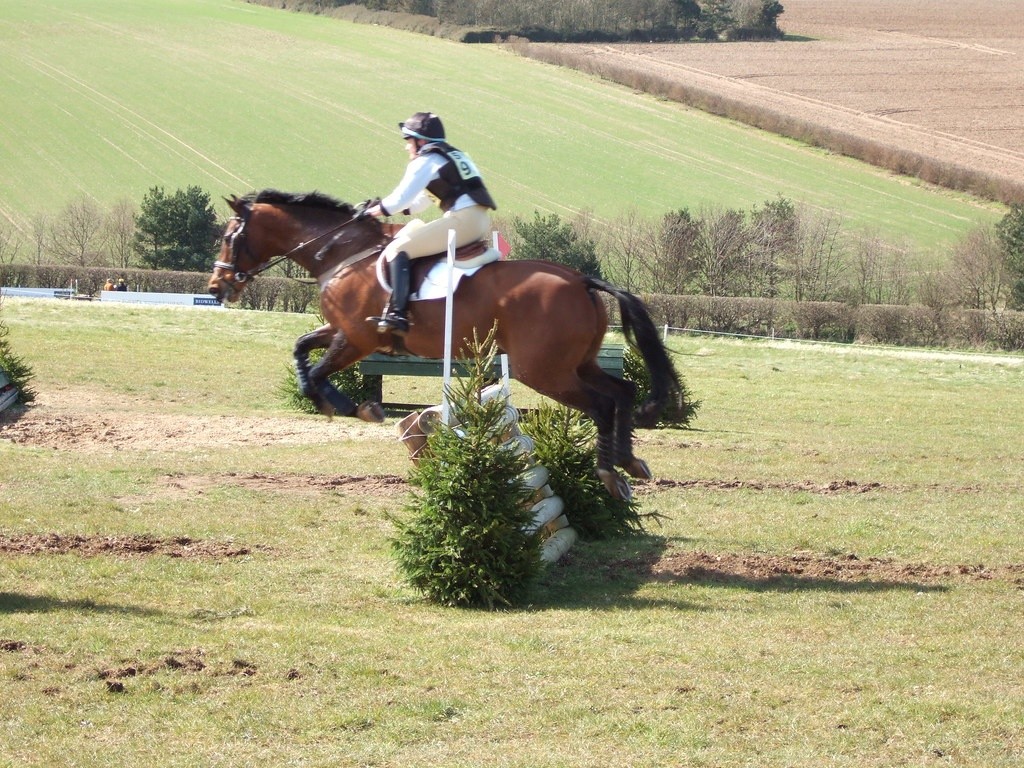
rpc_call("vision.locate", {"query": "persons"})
[117,279,127,291]
[104,279,117,291]
[353,112,497,335]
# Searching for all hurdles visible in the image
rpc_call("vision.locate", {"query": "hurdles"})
[393,228,580,567]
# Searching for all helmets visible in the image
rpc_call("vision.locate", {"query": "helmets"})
[403,112,444,141]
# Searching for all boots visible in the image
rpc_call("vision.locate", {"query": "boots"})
[365,251,409,337]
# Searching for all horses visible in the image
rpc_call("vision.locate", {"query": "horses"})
[208,190,685,501]
